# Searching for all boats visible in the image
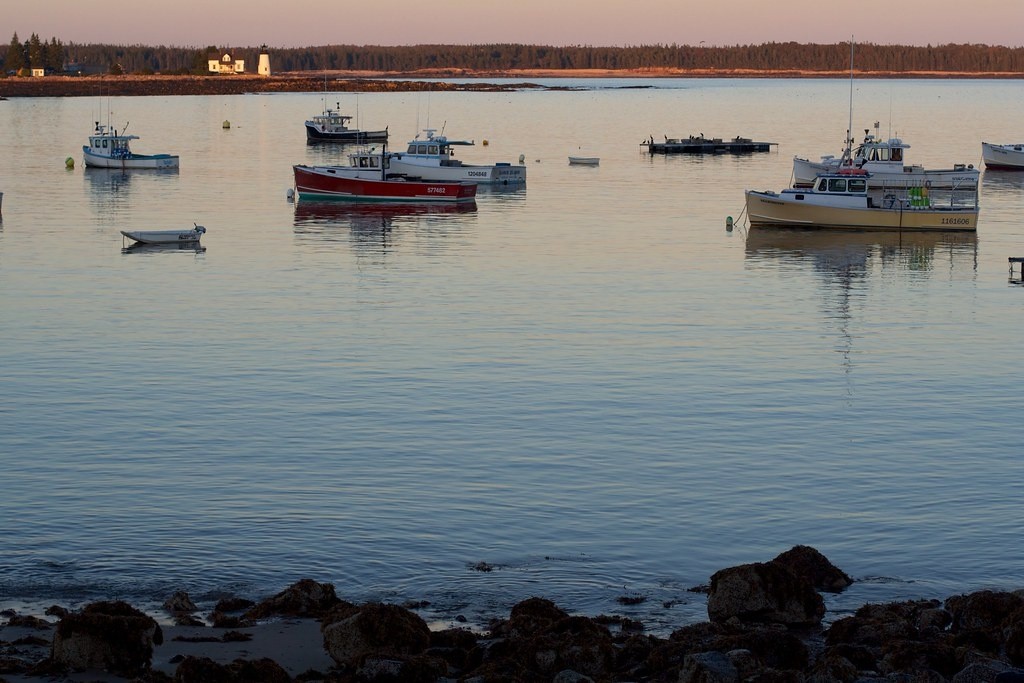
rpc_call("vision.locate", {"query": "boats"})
[567,145,601,164]
[793,95,980,192]
[291,81,478,206]
[120,222,207,243]
[305,68,390,144]
[744,33,981,233]
[310,71,527,186]
[80,69,181,171]
[980,141,1024,171]
[639,132,779,155]
